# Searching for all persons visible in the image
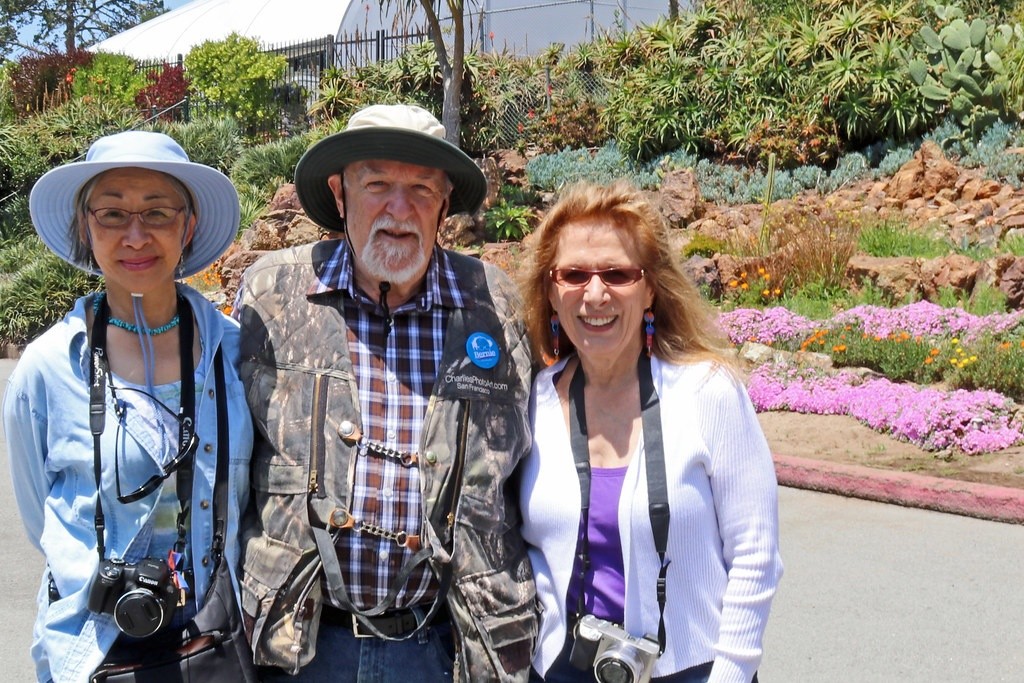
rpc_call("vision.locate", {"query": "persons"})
[231,103,547,682]
[518,181,785,683]
[2,133,252,683]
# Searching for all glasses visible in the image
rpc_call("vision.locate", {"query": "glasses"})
[84,203,185,228]
[549,265,645,287]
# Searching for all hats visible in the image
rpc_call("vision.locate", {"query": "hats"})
[294,104,486,232]
[29,131,239,279]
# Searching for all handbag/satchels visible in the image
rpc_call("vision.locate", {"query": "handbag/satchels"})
[89,556,262,683]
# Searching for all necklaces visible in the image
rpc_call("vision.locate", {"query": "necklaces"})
[92,291,179,335]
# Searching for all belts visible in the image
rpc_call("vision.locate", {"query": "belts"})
[321,602,450,638]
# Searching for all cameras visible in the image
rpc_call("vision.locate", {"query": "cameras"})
[88,558,181,639]
[570,613,660,683]
